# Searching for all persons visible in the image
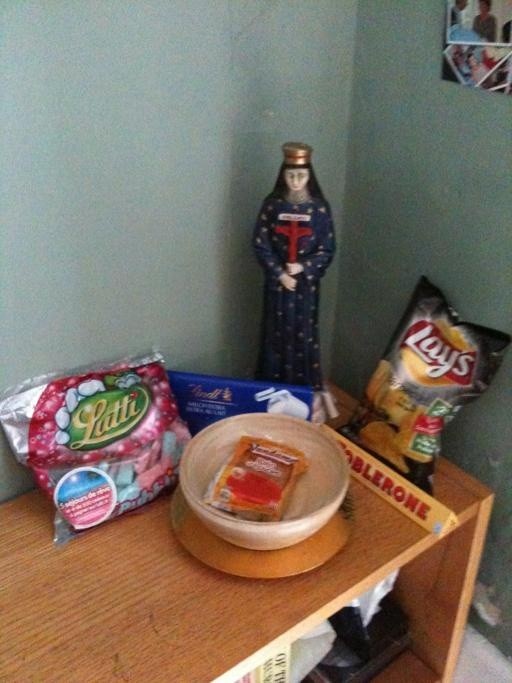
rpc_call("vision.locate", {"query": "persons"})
[472,0,498,43]
[452,41,508,90]
[451,0,469,26]
[249,140,338,391]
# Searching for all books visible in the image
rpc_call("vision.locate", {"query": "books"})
[308,594,416,682]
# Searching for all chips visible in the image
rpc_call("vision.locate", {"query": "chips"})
[359,320,475,474]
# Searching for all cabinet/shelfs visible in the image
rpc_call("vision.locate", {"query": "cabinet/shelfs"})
[0,381,495,683]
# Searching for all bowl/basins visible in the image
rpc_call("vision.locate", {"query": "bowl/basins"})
[175,410,353,552]
